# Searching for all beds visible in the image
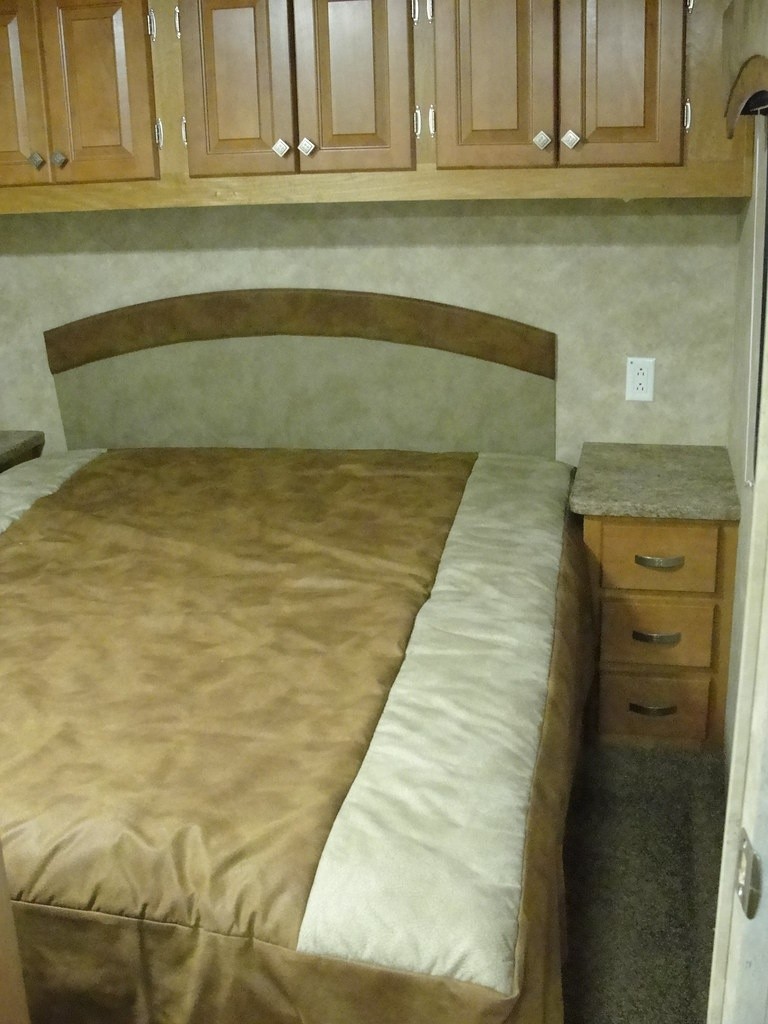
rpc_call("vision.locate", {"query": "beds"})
[0,288,590,1024]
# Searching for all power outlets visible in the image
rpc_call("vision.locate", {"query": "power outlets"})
[626,357,656,400]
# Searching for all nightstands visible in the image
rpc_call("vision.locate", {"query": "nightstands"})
[569,443,743,752]
[0,430,45,473]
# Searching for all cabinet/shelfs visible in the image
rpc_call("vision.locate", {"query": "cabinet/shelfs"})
[1,0,768,211]
